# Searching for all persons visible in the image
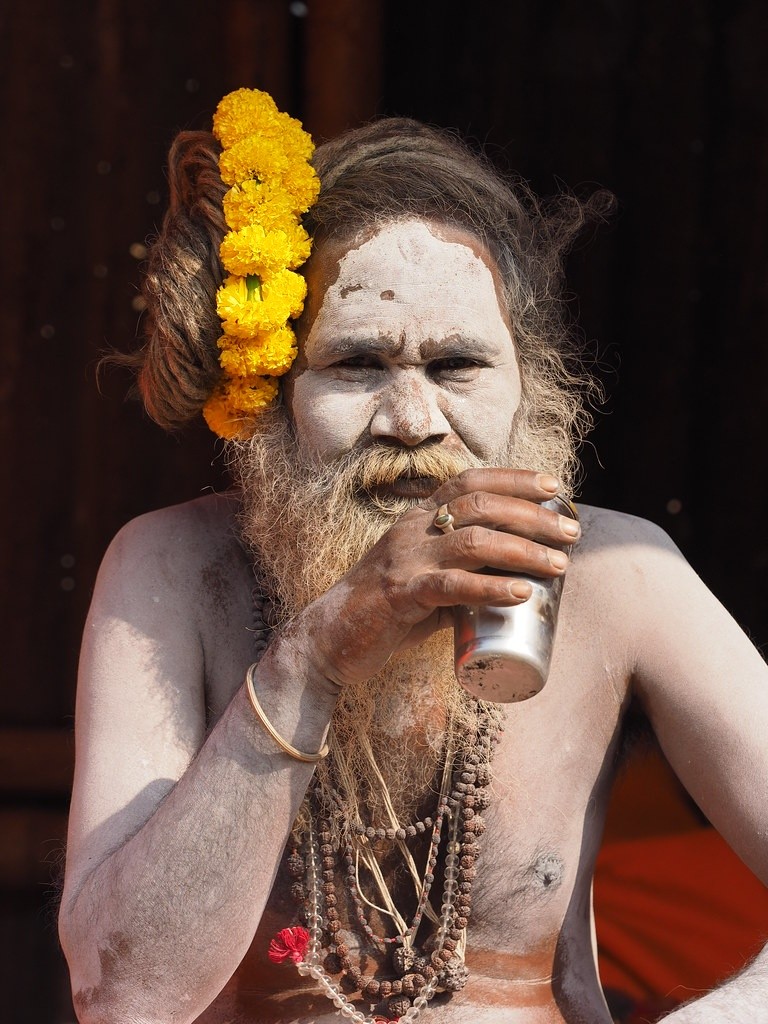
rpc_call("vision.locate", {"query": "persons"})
[57,88,768,1024]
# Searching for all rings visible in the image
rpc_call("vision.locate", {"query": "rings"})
[434,503,454,534]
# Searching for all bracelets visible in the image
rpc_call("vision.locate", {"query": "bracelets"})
[246,663,329,761]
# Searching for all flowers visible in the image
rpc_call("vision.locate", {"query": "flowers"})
[199,84,320,440]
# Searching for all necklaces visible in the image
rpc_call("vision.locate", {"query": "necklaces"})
[251,564,504,1024]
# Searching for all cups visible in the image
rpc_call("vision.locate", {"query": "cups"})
[455,491,581,702]
